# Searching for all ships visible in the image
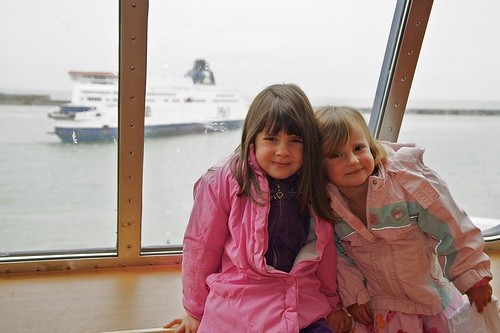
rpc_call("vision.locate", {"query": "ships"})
[48,57,258,144]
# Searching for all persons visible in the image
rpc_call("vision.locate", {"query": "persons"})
[163,85,349,333]
[313,107,493,333]
